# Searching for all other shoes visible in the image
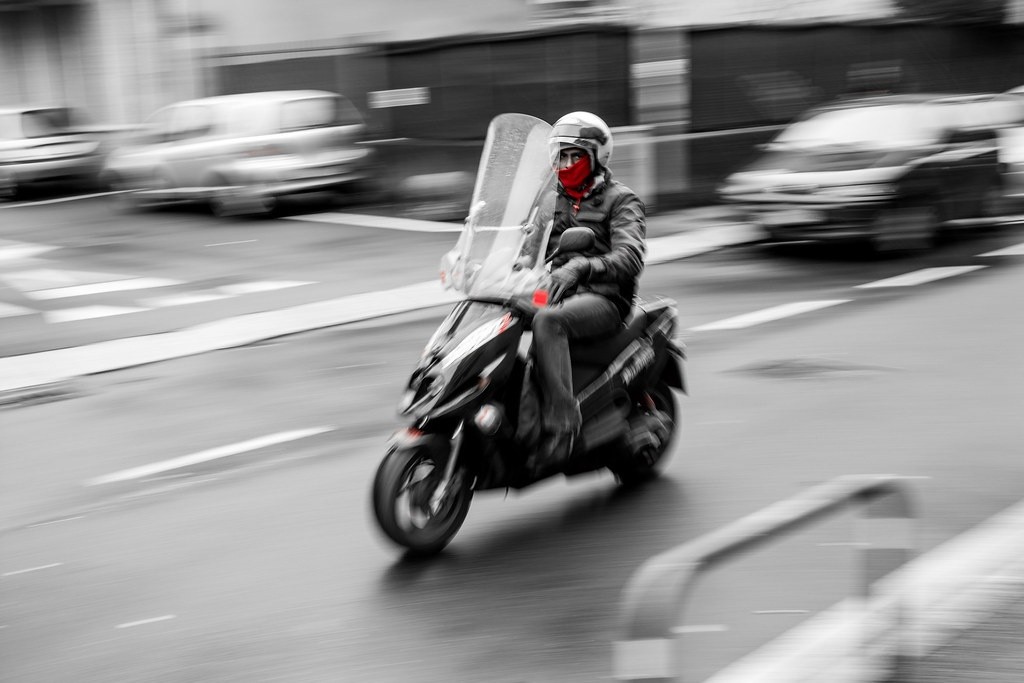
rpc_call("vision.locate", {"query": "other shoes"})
[528,428,574,472]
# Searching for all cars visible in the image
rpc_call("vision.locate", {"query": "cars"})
[0,109,108,196]
[716,91,1024,260]
[99,88,377,220]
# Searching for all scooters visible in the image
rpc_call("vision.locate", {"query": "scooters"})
[362,111,690,556]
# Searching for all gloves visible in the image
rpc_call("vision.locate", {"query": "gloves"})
[533,255,590,307]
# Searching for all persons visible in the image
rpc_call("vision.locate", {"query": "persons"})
[459,111,646,471]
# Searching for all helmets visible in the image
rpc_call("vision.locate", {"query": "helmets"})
[547,110,613,177]
